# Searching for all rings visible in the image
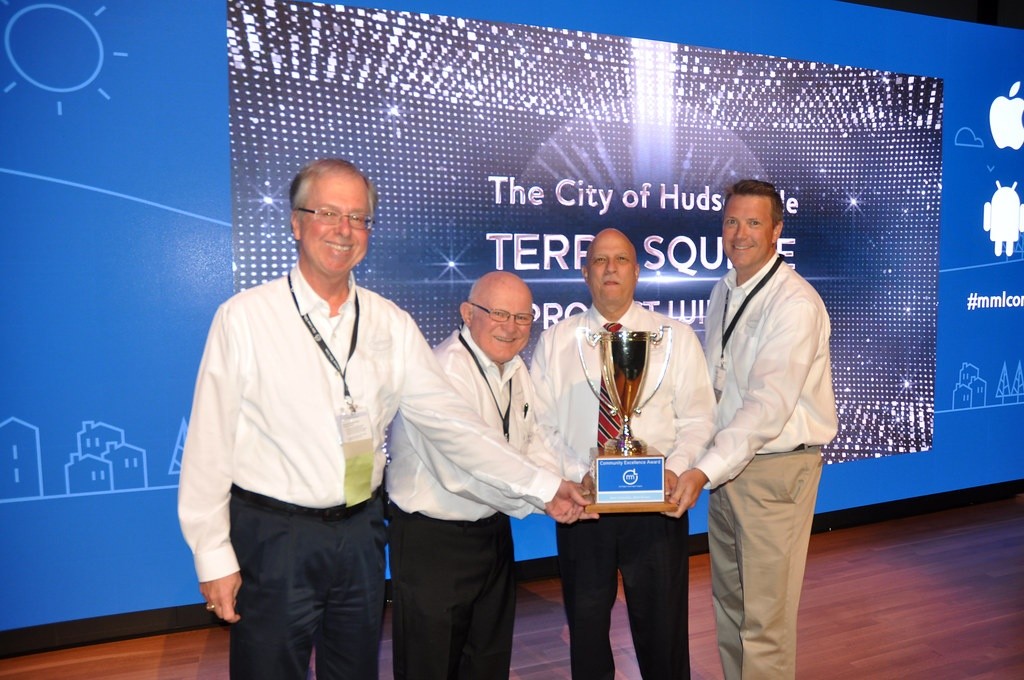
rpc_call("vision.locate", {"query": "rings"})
[206,604,216,612]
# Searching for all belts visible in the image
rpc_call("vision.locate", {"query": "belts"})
[792,444,813,450]
[390,501,503,527]
[231,484,381,523]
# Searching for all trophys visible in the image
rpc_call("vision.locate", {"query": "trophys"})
[573,323,681,516]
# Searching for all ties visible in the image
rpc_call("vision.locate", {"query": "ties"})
[597,322,625,457]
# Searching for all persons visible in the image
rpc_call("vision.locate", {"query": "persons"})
[174,156,842,680]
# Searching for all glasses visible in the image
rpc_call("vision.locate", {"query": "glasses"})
[470,302,535,326]
[296,207,376,230]
